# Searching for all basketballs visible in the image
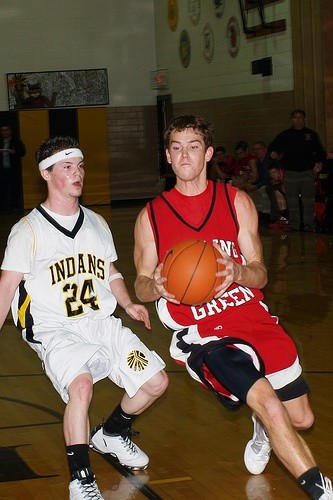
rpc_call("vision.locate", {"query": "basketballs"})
[160,239,225,306]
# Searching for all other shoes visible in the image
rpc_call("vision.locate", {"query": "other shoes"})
[283,224,300,231]
[304,224,315,232]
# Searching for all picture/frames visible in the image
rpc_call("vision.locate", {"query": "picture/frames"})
[7,69,110,110]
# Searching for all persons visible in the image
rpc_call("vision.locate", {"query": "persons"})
[212,110,333,232]
[0,136,169,500]
[134,116,333,499]
[0,125,26,215]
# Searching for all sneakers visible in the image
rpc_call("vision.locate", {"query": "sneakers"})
[243,412,273,475]
[269,216,291,228]
[90,423,150,471]
[315,471,333,500]
[68,468,105,500]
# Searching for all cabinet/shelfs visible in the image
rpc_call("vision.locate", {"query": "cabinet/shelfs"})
[0,107,112,209]
